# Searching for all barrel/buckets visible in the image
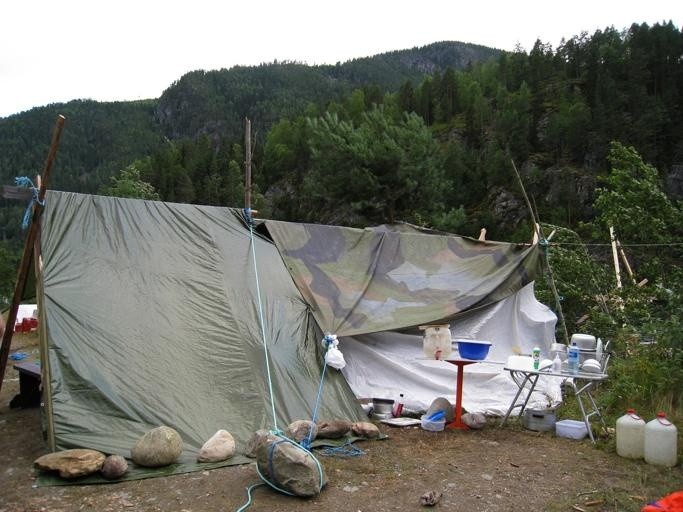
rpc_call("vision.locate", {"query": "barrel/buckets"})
[422,325,452,360]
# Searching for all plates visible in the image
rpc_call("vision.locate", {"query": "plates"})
[596,338,603,362]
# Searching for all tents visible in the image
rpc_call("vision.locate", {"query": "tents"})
[0,112,568,488]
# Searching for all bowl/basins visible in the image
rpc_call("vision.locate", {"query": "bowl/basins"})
[550,342,567,352]
[372,397,395,414]
[580,359,602,373]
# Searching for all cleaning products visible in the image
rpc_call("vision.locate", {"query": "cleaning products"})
[567,341,580,373]
[392,393,405,416]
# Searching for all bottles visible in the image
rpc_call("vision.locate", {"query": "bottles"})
[394,393,406,416]
[532,343,541,369]
[552,353,563,372]
[568,343,580,375]
[32,344,40,365]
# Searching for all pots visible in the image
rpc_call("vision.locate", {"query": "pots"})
[568,333,597,352]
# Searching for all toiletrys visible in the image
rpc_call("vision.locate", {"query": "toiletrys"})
[553,351,561,373]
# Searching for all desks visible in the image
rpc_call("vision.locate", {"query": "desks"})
[9,360,41,408]
[496,367,612,445]
[415,354,506,431]
